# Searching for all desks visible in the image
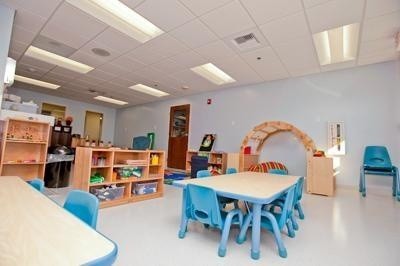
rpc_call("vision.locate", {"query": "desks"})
[173,171,303,259]
[0,176,118,266]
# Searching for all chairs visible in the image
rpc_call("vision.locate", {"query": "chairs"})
[179,167,304,259]
[359,146,400,201]
[31,178,99,230]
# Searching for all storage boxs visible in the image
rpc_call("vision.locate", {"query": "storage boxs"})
[90,182,160,202]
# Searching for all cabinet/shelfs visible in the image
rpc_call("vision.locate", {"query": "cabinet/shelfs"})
[72,146,165,209]
[0,117,51,183]
[186,151,228,176]
